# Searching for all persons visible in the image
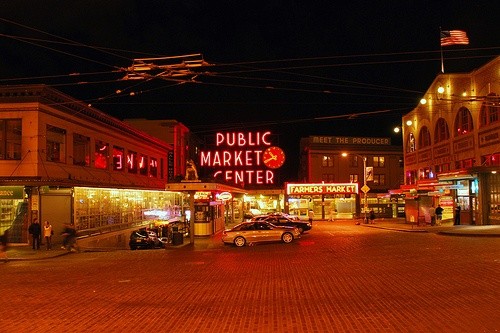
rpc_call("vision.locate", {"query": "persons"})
[453,203,461,225]
[430,204,435,226]
[29,218,77,252]
[369,208,375,224]
[308,208,314,225]
[436,205,444,226]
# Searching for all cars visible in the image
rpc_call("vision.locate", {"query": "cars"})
[262,215,312,234]
[222,221,301,247]
[245,216,268,225]
[130,229,161,248]
